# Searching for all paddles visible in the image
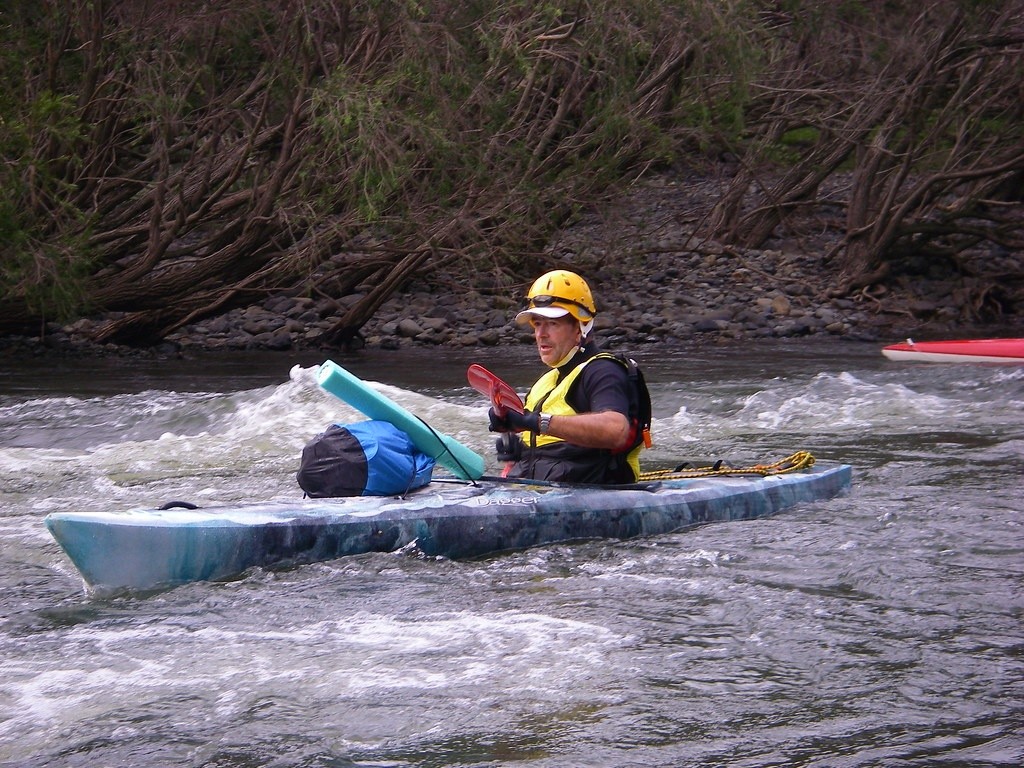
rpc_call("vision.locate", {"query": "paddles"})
[466,362,528,475]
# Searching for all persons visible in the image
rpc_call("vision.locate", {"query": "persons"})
[488,270,652,486]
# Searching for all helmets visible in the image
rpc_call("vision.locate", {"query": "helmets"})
[528,270,597,321]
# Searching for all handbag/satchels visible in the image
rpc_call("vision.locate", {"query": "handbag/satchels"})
[296,419,436,496]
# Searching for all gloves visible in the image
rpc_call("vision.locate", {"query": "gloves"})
[496,435,521,460]
[489,405,541,436]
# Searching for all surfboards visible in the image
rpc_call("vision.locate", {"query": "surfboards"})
[879,334,1023,365]
[40,462,850,600]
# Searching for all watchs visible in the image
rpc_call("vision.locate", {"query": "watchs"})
[538,410,553,436]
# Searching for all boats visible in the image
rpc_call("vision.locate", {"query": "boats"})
[44,452,854,601]
[881,338,1024,362]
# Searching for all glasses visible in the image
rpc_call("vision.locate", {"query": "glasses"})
[521,295,555,311]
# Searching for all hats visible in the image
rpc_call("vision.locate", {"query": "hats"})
[515,282,594,338]
